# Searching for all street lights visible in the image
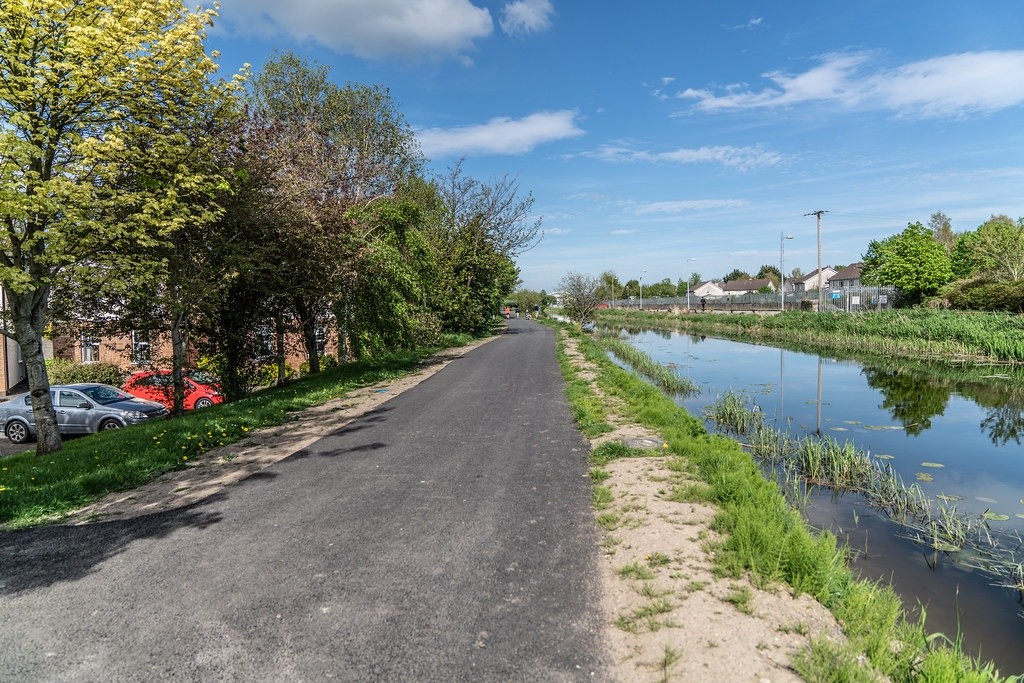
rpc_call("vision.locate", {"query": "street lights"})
[612,275,618,307]
[686,258,697,310]
[781,231,794,309]
[639,270,646,310]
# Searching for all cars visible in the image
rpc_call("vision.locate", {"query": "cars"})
[0,383,170,444]
[119,370,223,412]
